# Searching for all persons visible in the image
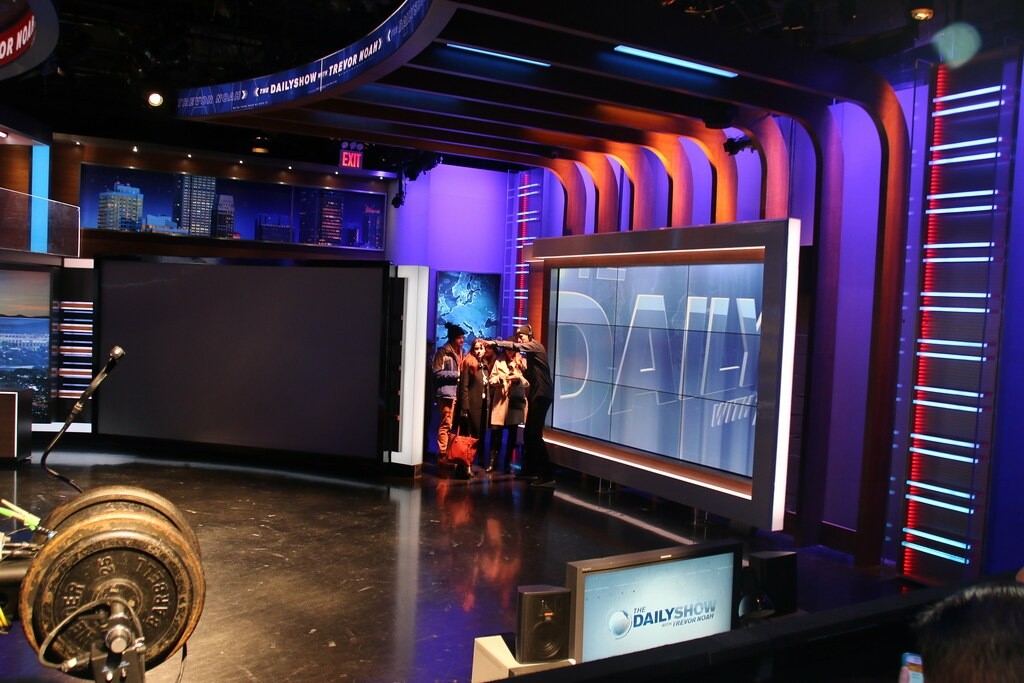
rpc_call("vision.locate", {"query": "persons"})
[898,584,1024,682]
[480,337,502,469]
[430,321,468,464]
[461,338,492,477]
[484,336,528,475]
[476,324,556,487]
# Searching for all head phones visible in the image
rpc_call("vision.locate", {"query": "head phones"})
[528,324,533,339]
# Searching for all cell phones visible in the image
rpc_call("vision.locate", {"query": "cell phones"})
[901,652,924,683]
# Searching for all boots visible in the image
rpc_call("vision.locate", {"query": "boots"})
[486,450,498,472]
[503,452,511,471]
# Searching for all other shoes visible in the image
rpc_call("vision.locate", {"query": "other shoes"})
[465,464,476,476]
[531,476,556,487]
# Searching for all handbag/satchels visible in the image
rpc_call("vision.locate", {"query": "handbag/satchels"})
[447,415,479,466]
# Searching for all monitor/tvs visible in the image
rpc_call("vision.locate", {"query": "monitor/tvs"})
[565,539,743,664]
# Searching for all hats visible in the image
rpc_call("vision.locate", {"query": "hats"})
[515,326,529,337]
[445,322,464,339]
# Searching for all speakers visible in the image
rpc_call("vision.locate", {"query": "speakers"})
[749,551,799,620]
[515,584,572,665]
